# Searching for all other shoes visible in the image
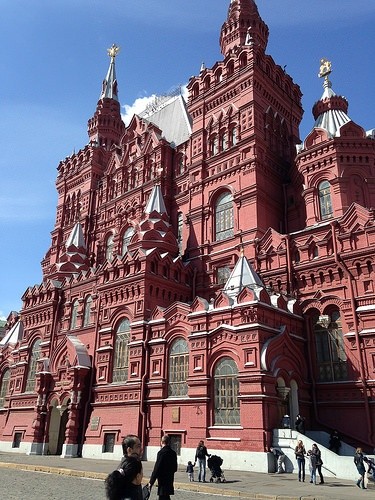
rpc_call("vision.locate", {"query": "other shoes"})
[299,479,301,481]
[203,480,206,483]
[313,481,316,485]
[276,472,281,474]
[303,479,304,482]
[190,480,194,482]
[310,481,313,483]
[199,480,202,482]
[319,482,324,484]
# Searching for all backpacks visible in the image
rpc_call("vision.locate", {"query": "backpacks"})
[197,446,206,457]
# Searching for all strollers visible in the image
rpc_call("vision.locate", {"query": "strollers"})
[207,455,227,483]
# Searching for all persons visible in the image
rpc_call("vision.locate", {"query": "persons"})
[186,461,196,482]
[307,443,324,484]
[104,457,144,500]
[294,440,306,483]
[354,448,370,489]
[149,436,177,500]
[195,440,211,483]
[116,434,144,499]
[270,446,286,474]
[307,450,318,486]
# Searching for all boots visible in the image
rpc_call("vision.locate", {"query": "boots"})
[361,482,367,489]
[357,480,361,488]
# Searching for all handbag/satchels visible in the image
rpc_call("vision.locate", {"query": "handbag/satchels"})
[143,484,151,500]
